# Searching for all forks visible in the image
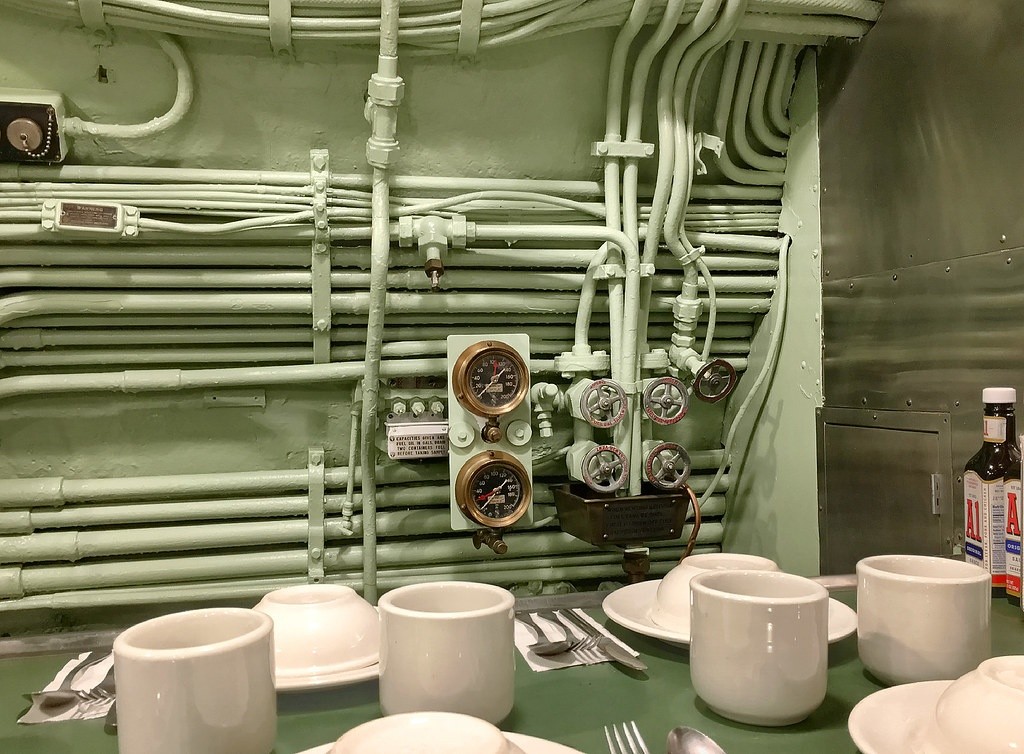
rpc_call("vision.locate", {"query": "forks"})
[536,609,602,651]
[602,719,650,754]
[74,665,115,702]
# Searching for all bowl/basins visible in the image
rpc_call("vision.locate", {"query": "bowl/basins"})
[249,583,382,679]
[909,655,1024,754]
[325,710,526,754]
[648,552,783,632]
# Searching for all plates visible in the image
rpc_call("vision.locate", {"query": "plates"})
[267,604,380,692]
[294,731,586,754]
[847,680,955,754]
[600,578,858,648]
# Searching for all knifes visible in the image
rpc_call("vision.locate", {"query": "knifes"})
[106,698,118,728]
[559,607,649,671]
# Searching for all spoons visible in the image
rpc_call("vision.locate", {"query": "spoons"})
[21,645,112,706]
[513,611,573,654]
[665,725,727,754]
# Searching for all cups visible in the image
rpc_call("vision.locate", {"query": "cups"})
[855,554,993,684]
[111,606,279,754]
[689,569,830,730]
[377,579,515,727]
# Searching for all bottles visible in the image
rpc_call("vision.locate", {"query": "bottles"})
[1004,435,1024,605]
[962,386,1021,598]
